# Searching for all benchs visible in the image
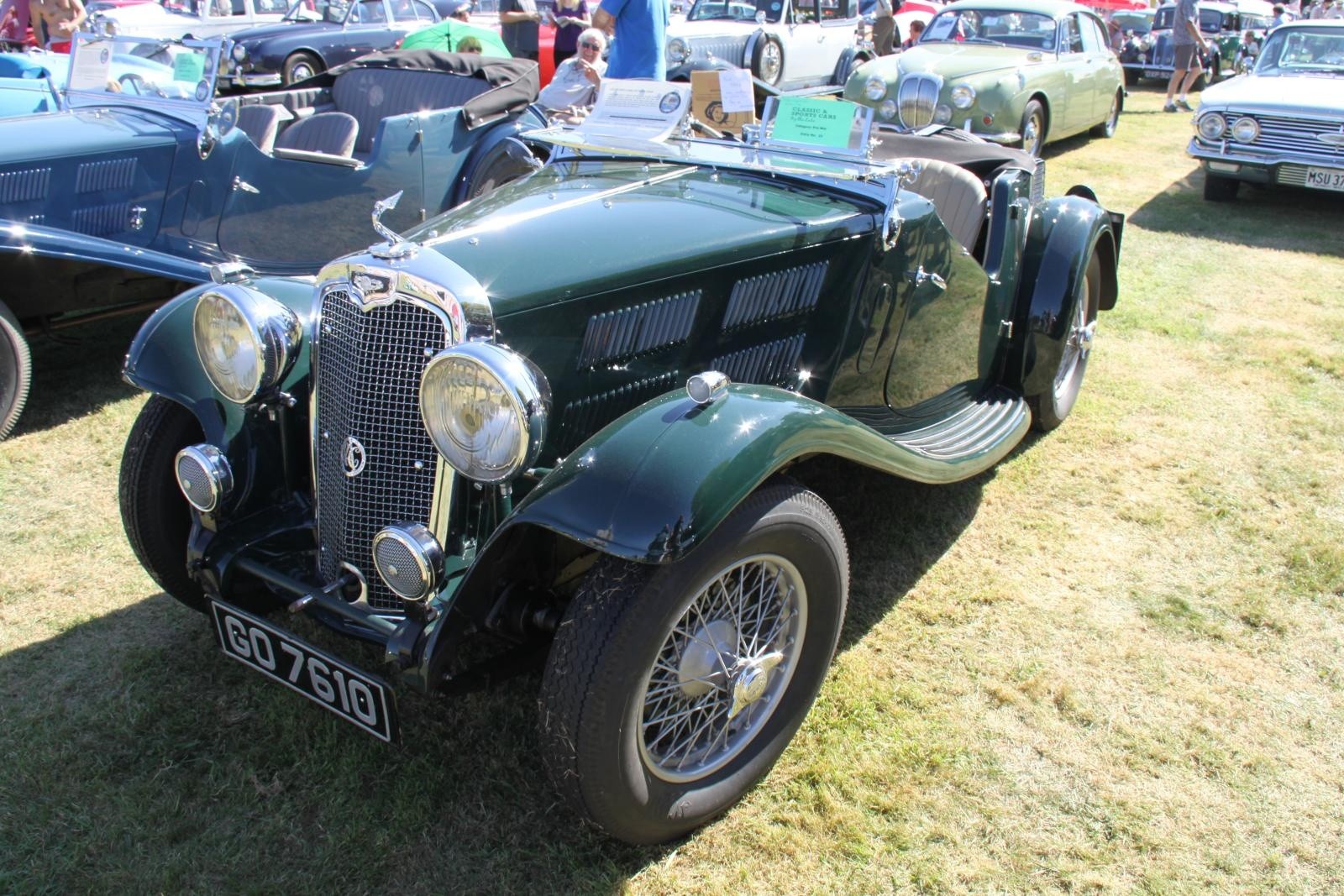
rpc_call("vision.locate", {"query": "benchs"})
[985,34,1056,46]
[774,127,1012,166]
[291,70,502,155]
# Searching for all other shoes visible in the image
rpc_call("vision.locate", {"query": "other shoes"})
[1164,104,1179,112]
[1176,99,1194,111]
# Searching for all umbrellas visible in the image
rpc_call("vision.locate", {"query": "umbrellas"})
[400,18,512,58]
[893,0,966,44]
[1076,0,1146,11]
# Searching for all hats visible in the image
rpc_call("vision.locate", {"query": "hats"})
[1108,19,1120,30]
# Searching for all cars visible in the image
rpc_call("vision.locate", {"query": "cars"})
[842,0,1128,159]
[118,95,1124,845]
[1104,0,1301,91]
[1186,19,1344,201]
[0,0,877,438]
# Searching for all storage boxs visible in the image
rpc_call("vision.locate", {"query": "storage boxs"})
[690,69,764,138]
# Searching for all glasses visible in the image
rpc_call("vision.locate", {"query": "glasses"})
[460,47,483,53]
[581,42,600,52]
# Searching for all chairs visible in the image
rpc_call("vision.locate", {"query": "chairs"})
[231,104,277,154]
[278,111,360,161]
[876,160,990,261]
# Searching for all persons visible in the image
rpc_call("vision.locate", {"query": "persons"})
[909,20,926,41]
[456,36,482,54]
[29,0,87,54]
[1245,30,1257,55]
[451,7,470,22]
[1108,20,1124,51]
[1301,0,1344,19]
[497,0,541,62]
[873,0,896,57]
[535,29,608,111]
[1266,6,1284,29]
[592,0,670,82]
[550,0,592,68]
[1164,0,1209,112]
